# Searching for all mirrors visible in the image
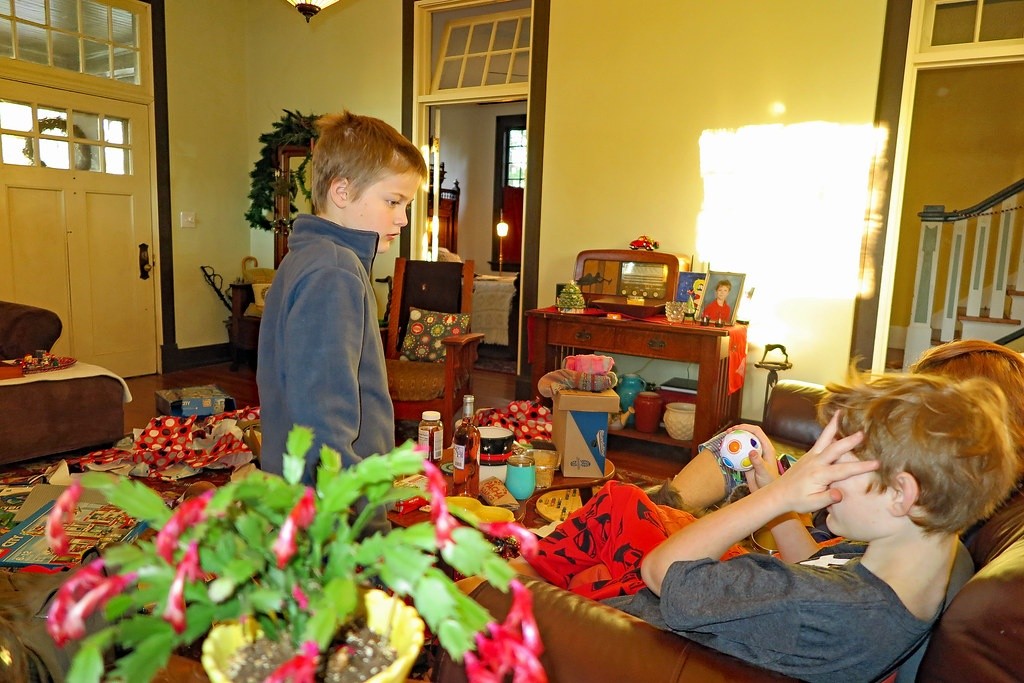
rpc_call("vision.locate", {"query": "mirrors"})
[274,144,312,270]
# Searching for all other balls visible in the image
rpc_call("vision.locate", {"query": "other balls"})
[720,429,763,472]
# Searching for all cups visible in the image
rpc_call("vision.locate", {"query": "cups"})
[528,449,558,489]
[634,392,662,433]
[665,301,687,324]
[505,454,536,501]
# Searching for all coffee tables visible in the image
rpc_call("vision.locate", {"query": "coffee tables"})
[352,445,616,580]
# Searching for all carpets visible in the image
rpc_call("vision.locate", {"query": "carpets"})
[474,357,516,374]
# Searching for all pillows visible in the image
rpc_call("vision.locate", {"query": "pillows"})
[251,283,271,306]
[400,306,470,363]
[428,247,461,263]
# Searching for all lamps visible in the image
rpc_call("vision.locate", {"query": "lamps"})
[496,208,509,275]
[286,0,342,23]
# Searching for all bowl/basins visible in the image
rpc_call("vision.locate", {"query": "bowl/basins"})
[477,427,515,466]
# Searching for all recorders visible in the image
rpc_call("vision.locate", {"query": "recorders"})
[573,250,679,307]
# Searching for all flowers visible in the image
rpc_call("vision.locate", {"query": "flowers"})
[43,426,548,683]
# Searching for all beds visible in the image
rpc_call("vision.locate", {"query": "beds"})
[424,162,520,345]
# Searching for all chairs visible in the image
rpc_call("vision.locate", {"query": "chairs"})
[230,268,275,371]
[384,257,486,449]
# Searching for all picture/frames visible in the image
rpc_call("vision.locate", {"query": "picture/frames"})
[695,270,746,325]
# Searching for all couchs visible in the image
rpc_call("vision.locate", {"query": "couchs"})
[430,378,1024,683]
[0,299,133,466]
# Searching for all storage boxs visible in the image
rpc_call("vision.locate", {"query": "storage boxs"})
[552,388,620,478]
[154,383,237,417]
[393,474,432,515]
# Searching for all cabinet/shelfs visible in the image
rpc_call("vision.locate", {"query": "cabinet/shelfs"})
[524,306,747,461]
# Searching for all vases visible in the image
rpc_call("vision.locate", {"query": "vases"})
[617,372,646,423]
[200,587,426,683]
[635,391,663,432]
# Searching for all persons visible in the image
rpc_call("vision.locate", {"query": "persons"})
[450,356,1020,683]
[256,107,427,591]
[648,338,1023,550]
[701,280,732,322]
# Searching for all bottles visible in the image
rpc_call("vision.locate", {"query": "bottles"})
[663,402,696,441]
[452,394,481,500]
[616,373,647,427]
[418,411,444,467]
